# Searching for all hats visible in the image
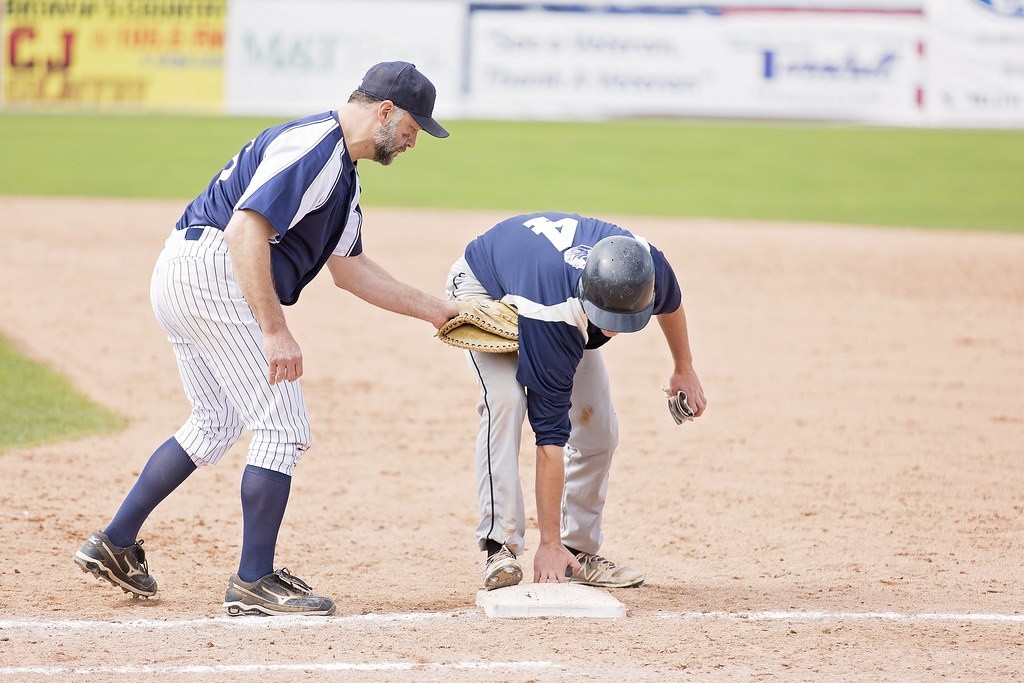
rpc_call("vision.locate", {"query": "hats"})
[358,61,450,138]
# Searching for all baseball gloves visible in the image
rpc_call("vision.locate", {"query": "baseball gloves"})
[434,298,520,354]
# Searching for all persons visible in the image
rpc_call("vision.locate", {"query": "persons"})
[447,211,708,592]
[75,61,460,617]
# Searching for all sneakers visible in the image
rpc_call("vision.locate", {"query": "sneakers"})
[73,529,158,600]
[539,553,644,588]
[483,545,523,589]
[223,567,336,617]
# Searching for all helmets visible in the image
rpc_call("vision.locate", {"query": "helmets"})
[580,235,654,332]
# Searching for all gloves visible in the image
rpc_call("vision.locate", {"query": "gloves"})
[662,384,694,426]
